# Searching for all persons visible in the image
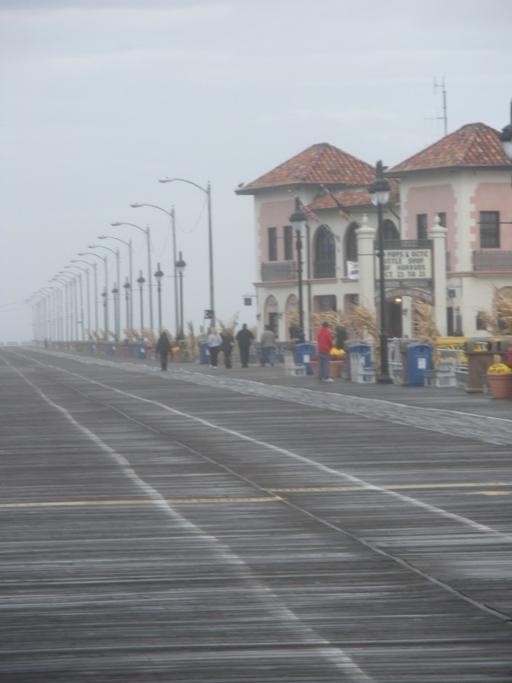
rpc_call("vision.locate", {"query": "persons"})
[219,328,234,367]
[154,330,174,370]
[316,321,336,382]
[206,327,222,369]
[235,322,256,368]
[259,323,277,368]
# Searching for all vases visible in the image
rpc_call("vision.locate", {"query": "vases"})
[486,376,512,399]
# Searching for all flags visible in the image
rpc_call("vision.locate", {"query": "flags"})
[293,196,319,222]
[324,187,352,223]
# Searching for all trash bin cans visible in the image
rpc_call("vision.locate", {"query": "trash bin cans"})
[198,341,210,364]
[138,344,145,358]
[295,343,316,374]
[348,342,376,385]
[407,343,432,385]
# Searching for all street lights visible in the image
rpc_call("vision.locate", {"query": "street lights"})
[288,194,307,344]
[27,177,214,342]
[367,160,396,382]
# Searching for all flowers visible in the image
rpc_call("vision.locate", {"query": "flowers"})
[486,363,512,377]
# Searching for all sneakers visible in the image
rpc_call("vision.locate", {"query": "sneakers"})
[209,363,274,368]
[319,378,333,383]
